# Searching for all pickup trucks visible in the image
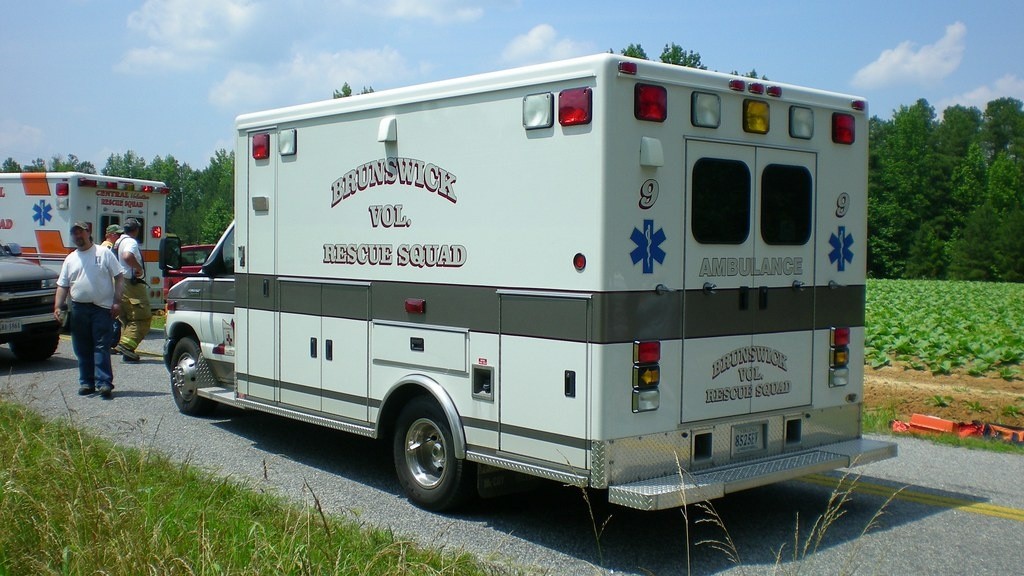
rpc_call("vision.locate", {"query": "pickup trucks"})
[163,243,217,303]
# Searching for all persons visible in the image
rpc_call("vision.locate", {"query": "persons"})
[54,221,124,394]
[102,217,152,361]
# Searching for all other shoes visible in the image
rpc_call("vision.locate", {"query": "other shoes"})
[99,385,111,396]
[79,387,95,395]
[114,345,140,361]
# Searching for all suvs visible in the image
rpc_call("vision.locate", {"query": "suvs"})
[0,242,68,363]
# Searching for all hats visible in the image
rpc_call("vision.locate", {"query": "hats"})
[71,221,88,230]
[124,218,141,232]
[106,224,124,234]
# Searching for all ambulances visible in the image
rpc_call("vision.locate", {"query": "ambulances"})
[1,171,167,313]
[157,52,898,516]
